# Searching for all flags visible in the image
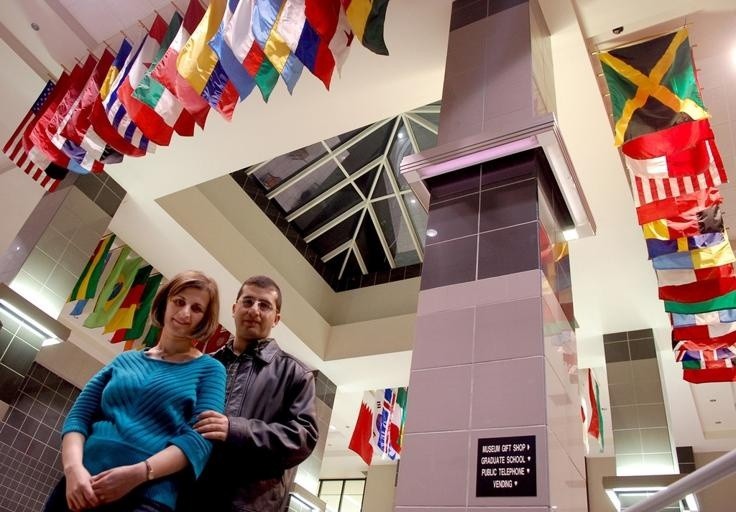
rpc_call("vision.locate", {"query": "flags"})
[1,0,389,193]
[539,224,578,383]
[598,25,736,384]
[578,369,603,456]
[348,387,406,465]
[66,233,235,356]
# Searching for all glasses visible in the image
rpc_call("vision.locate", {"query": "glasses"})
[236,298,278,312]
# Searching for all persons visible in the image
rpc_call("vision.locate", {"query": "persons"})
[192,275,320,512]
[41,271,227,512]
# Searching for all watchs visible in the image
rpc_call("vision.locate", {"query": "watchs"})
[144,461,154,480]
